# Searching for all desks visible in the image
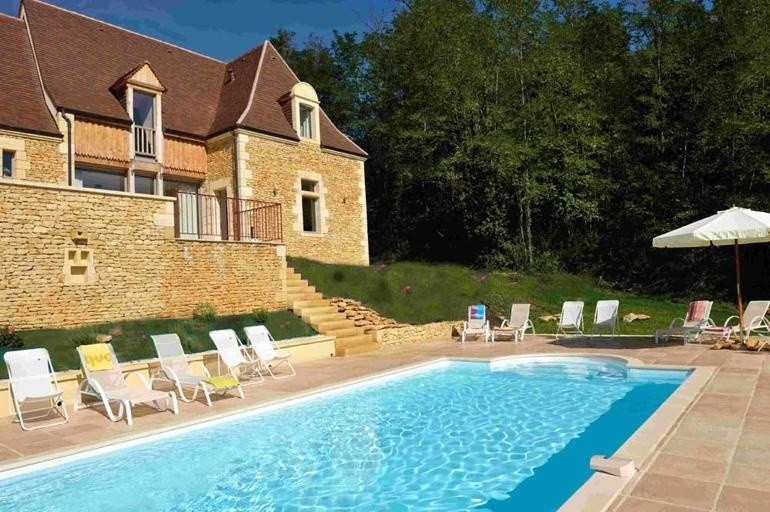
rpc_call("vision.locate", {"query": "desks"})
[700,327,738,344]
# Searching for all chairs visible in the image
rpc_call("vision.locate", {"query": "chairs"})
[150,333,245,406]
[462,305,490,343]
[555,301,585,340]
[590,300,621,340]
[719,301,770,345]
[3,347,69,431]
[73,341,179,424]
[208,328,265,386]
[655,300,717,346]
[243,325,296,380]
[492,304,536,343]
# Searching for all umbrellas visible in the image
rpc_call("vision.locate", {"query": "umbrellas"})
[653,203,770,328]
